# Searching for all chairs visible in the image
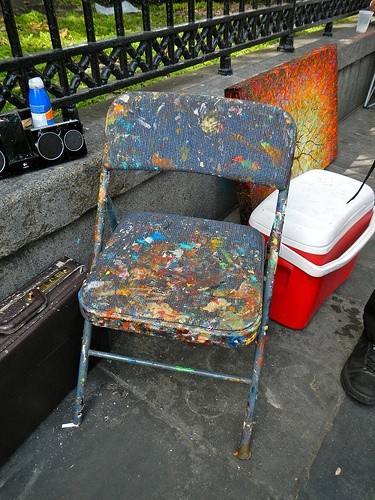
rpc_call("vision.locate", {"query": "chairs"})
[71,90,298,462]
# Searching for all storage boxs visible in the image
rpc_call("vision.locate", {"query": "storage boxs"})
[244,168,375,331]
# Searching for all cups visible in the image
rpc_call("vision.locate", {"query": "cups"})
[355,10,374,34]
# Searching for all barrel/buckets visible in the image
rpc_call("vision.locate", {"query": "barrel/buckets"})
[247,168,375,330]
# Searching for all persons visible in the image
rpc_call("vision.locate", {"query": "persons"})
[340,289,375,407]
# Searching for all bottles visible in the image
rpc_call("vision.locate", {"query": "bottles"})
[27,75,57,128]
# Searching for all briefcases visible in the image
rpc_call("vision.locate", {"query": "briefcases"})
[0,254,110,468]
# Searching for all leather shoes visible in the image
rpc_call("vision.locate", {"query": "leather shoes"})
[339,331,375,404]
